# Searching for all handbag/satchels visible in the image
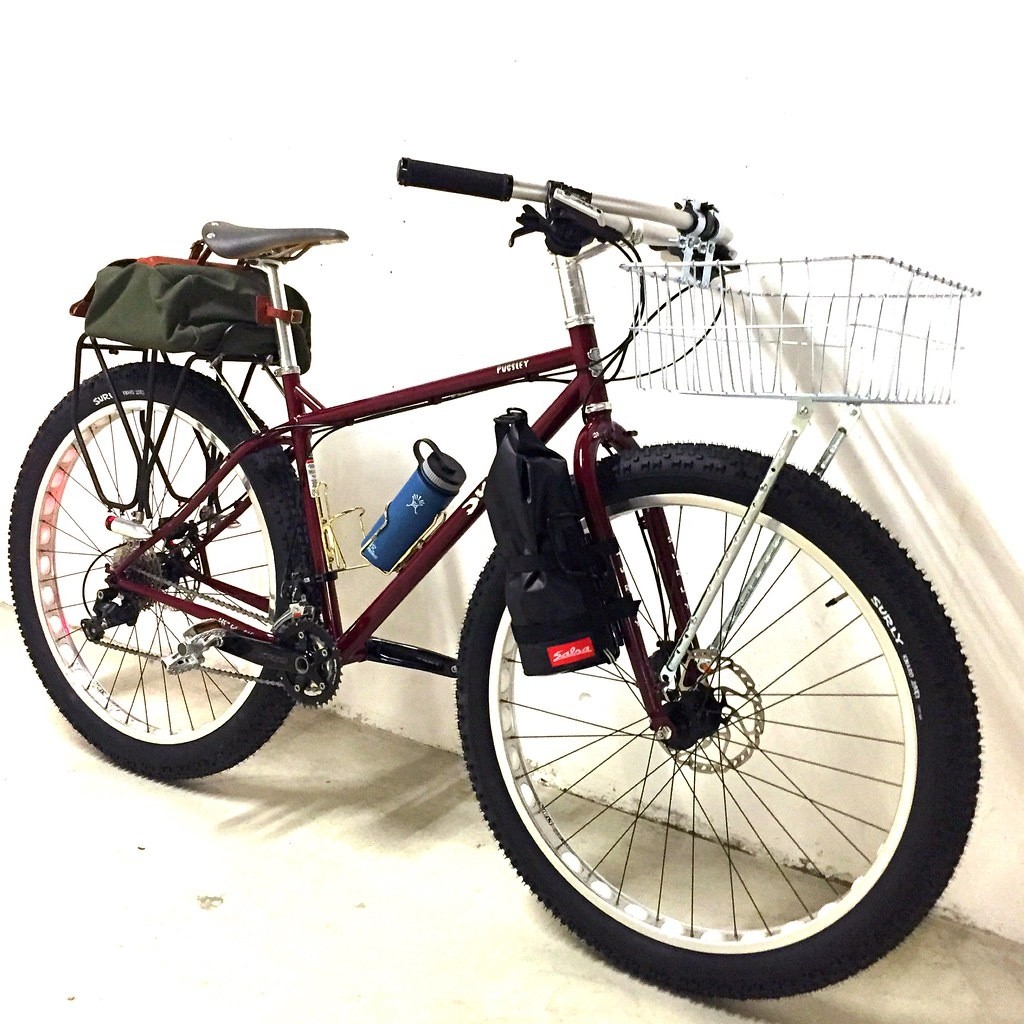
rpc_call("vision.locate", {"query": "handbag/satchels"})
[71,257,312,375]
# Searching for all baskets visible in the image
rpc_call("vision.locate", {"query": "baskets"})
[620,255,982,405]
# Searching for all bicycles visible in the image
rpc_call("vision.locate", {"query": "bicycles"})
[8,155,983,1005]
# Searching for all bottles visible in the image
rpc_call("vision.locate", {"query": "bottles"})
[360,438,466,571]
